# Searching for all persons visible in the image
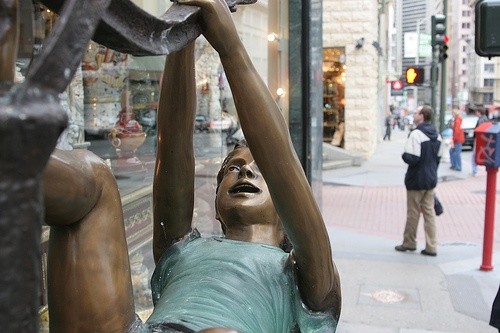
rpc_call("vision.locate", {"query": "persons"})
[383,101,500,140]
[0,0,342,333]
[394,103,443,256]
[470,106,489,177]
[449,107,465,170]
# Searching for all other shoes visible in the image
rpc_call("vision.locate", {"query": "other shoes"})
[421,249,436,255]
[395,244,417,252]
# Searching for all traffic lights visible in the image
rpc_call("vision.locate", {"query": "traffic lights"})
[432,13,447,45]
[406,68,424,84]
[438,45,448,63]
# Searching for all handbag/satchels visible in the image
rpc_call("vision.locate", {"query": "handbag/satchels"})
[434,195,444,216]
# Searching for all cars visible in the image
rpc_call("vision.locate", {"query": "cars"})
[458,115,480,149]
